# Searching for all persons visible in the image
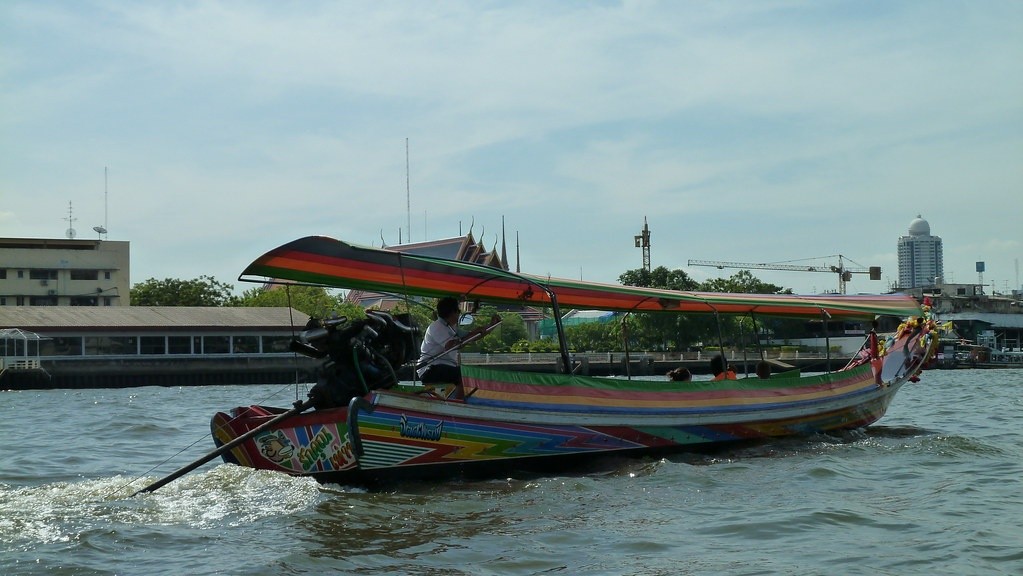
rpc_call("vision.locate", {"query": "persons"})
[756,362,770,379]
[709,355,737,381]
[416,298,501,400]
[667,368,692,382]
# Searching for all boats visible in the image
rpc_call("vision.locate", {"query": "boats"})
[209,234,940,492]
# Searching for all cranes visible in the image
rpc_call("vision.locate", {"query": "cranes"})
[688,254,884,296]
